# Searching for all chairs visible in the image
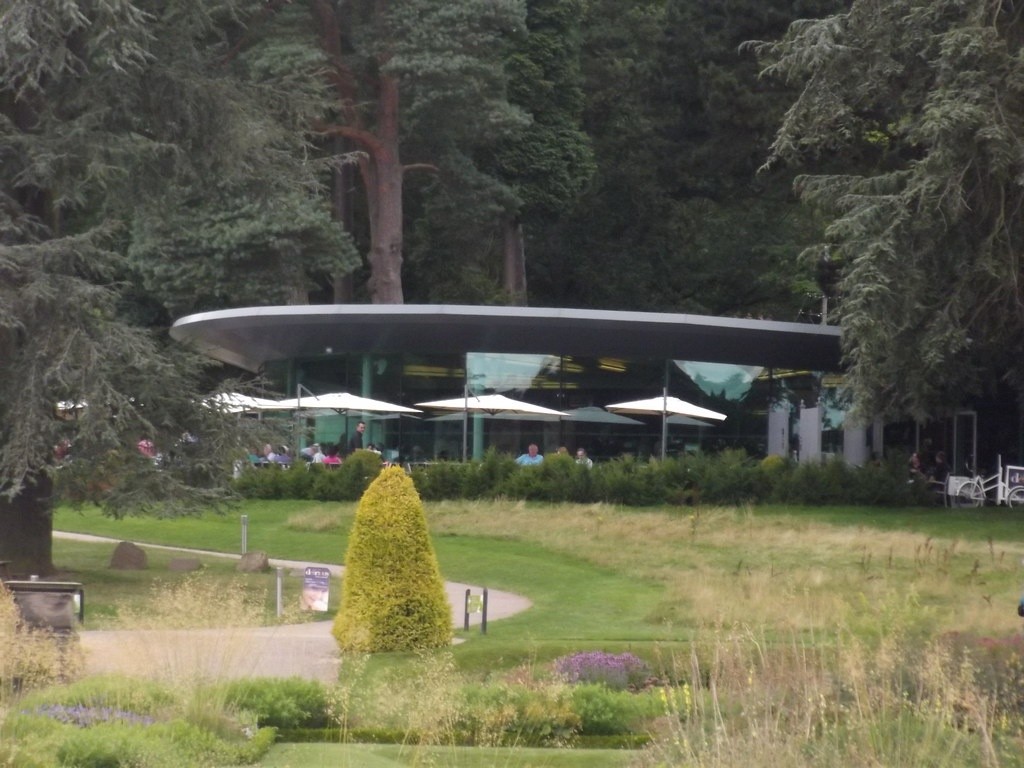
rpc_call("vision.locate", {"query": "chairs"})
[254,461,413,474]
[926,472,951,509]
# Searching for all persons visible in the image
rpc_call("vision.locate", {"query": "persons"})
[349,422,365,454]
[515,444,544,465]
[908,453,924,478]
[244,443,343,463]
[575,447,594,468]
[928,452,948,490]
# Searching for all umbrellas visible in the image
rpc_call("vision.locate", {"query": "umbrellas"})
[256,392,423,414]
[519,406,645,425]
[295,409,379,419]
[665,414,715,427]
[198,392,276,414]
[426,413,560,424]
[605,395,728,422]
[371,414,422,421]
[412,393,572,419]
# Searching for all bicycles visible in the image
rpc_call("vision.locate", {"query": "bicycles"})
[956,466,1024,511]
